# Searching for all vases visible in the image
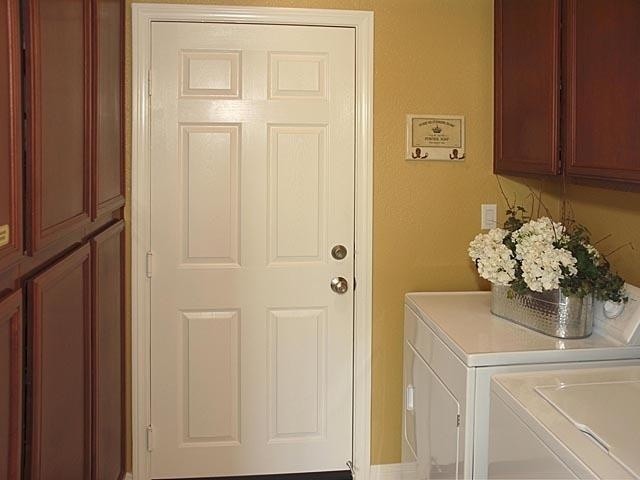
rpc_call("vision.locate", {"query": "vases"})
[490,283,593,338]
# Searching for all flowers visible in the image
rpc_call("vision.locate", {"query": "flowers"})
[467,169,633,303]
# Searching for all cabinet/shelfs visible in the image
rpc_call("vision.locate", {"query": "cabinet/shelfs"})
[493,0,640,184]
